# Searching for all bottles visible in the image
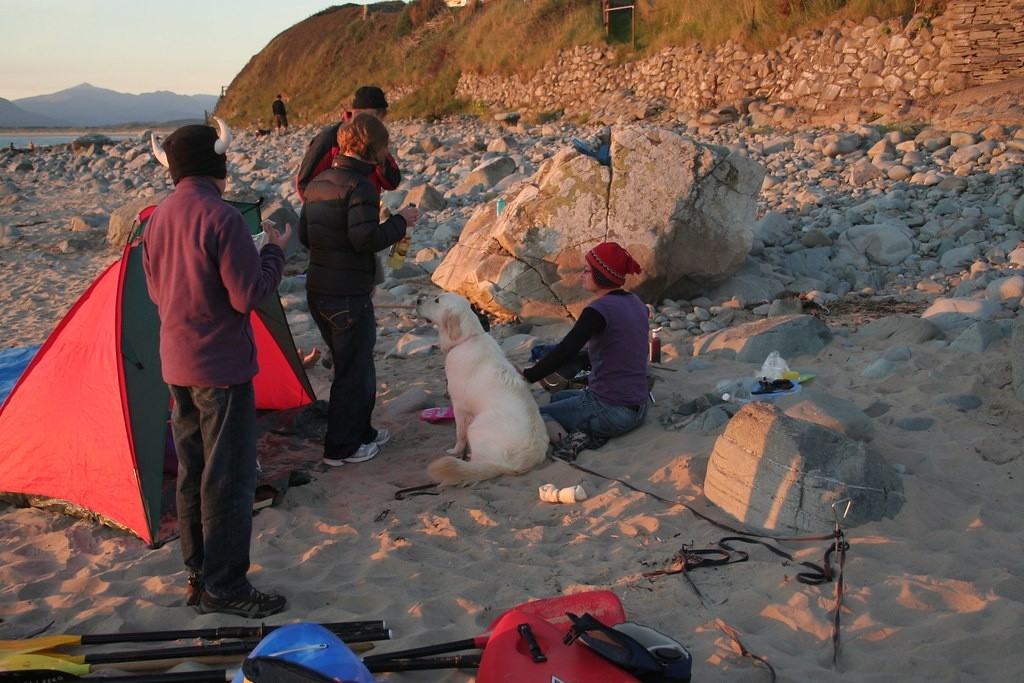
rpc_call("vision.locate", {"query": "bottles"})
[385,202,417,270]
[649,329,661,365]
[496,198,506,219]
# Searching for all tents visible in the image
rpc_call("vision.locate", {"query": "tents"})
[0,197,330,551]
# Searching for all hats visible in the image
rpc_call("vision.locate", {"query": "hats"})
[151,116,231,181]
[352,87,388,109]
[585,242,642,289]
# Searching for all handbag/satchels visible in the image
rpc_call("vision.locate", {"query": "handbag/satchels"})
[760,351,790,383]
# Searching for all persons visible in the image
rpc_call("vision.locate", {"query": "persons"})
[272,95,288,137]
[512,241,649,461]
[141,115,291,618]
[295,86,401,382]
[298,114,418,466]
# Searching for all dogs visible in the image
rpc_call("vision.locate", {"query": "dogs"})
[415,293,548,486]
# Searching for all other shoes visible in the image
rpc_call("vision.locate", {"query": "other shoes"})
[547,431,591,464]
[420,407,455,422]
[587,436,610,450]
[751,378,792,394]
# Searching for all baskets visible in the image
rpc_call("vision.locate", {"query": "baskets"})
[535,359,587,394]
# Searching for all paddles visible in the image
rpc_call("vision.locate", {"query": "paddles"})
[0,590,630,683]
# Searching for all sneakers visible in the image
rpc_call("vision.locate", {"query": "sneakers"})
[322,442,379,467]
[186,573,205,605]
[371,428,391,446]
[201,583,286,618]
